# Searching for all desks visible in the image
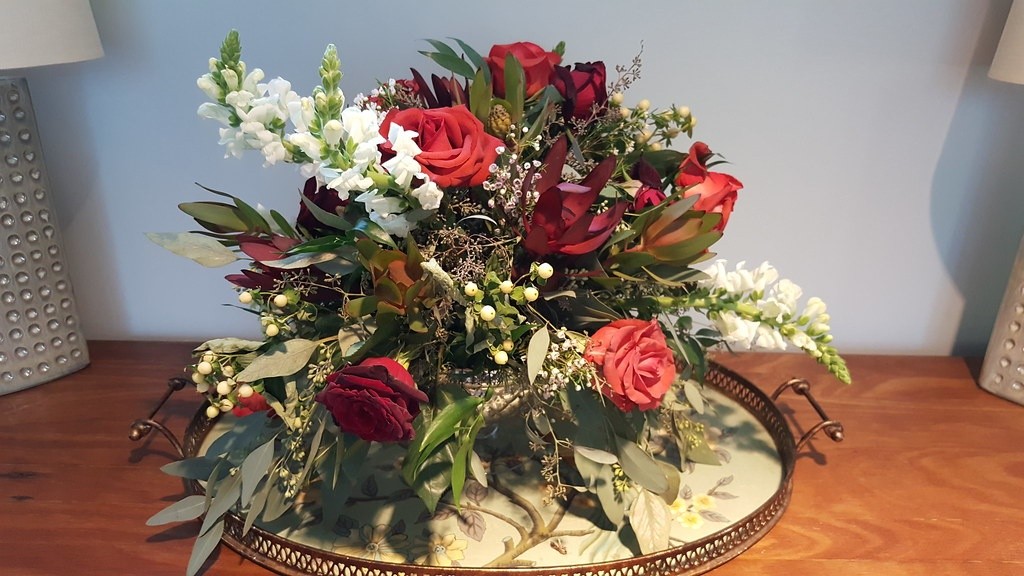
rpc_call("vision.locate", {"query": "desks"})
[0,333,1024,576]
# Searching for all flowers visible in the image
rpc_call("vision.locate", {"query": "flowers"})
[153,41,856,552]
